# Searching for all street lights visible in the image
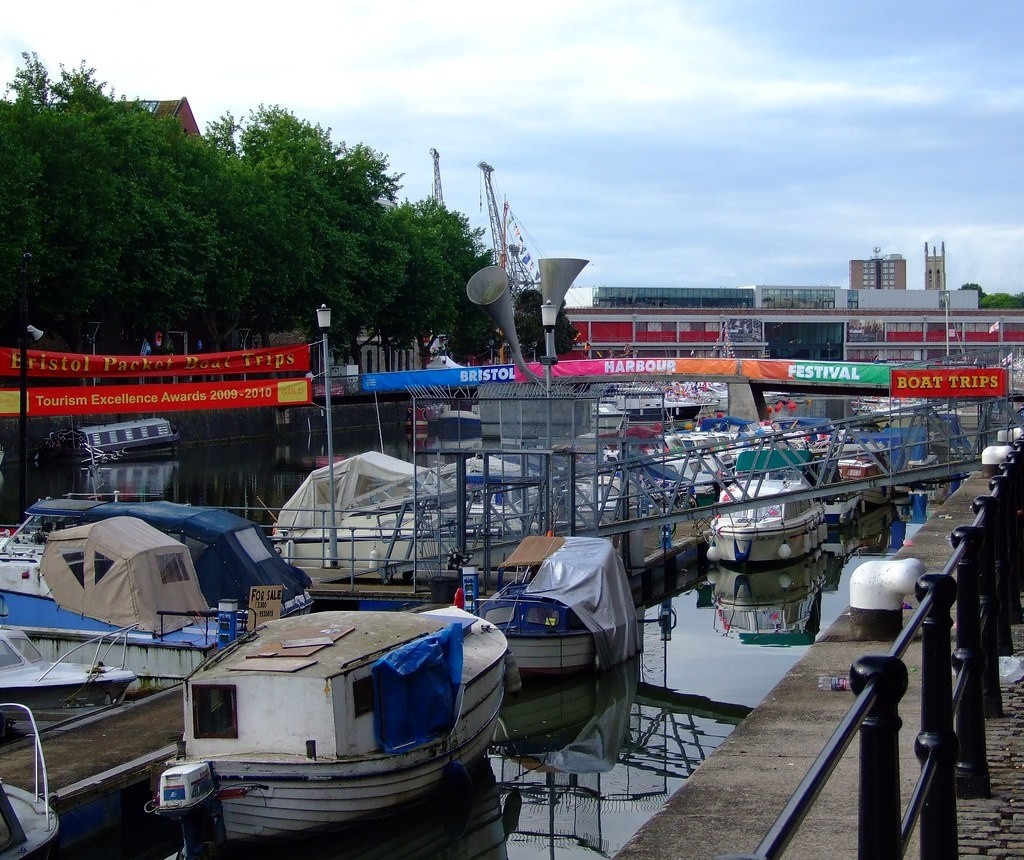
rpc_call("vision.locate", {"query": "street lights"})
[317,303,343,569]
[540,299,558,450]
[20,252,44,523]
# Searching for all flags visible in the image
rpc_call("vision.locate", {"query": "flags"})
[1002,353,1012,365]
[574,330,631,358]
[990,321,999,333]
[507,215,540,280]
[710,322,735,358]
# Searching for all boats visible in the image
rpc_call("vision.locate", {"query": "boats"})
[63,418,181,457]
[0,703,61,860]
[0,497,314,693]
[145,606,522,860]
[707,502,901,647]
[473,530,642,675]
[73,440,180,497]
[0,625,138,709]
[492,656,640,772]
[193,758,522,859]
[272,382,894,567]
